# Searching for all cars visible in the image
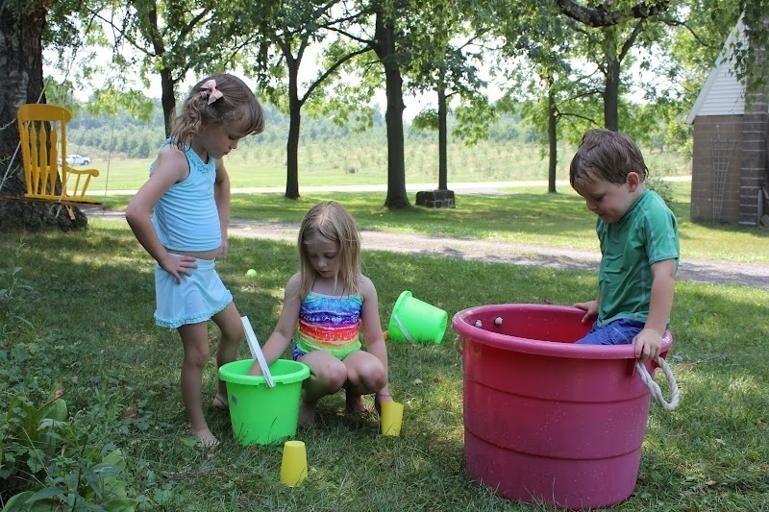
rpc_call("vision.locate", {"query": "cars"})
[58,155,91,168]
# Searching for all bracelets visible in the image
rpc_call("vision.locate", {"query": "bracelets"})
[376,393,390,397]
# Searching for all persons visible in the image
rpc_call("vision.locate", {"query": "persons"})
[568,127,681,361]
[124,74,265,453]
[248,198,396,427]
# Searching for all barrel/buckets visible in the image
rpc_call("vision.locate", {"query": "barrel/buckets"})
[452,303,680,510]
[217,314,312,447]
[387,291,449,346]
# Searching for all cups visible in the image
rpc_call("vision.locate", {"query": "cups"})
[280,441,308,488]
[380,400,405,437]
